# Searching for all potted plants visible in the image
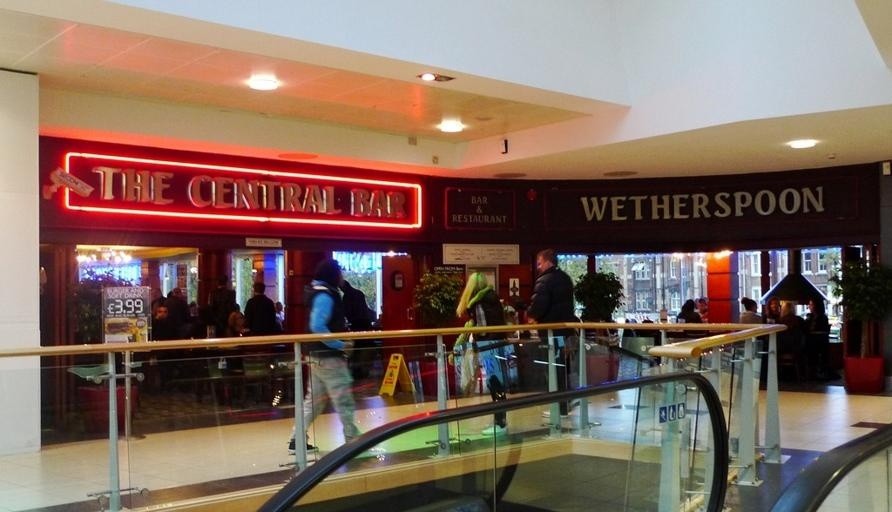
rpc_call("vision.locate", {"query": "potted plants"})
[827,255,891,399]
[69,265,143,435]
[405,270,465,397]
[574,271,627,391]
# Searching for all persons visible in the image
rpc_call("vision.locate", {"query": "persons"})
[456,271,512,435]
[287,258,365,453]
[675,297,830,383]
[148,273,382,386]
[524,247,575,420]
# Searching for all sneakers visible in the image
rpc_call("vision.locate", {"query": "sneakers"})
[542,411,570,418]
[481,425,508,435]
[289,439,318,451]
